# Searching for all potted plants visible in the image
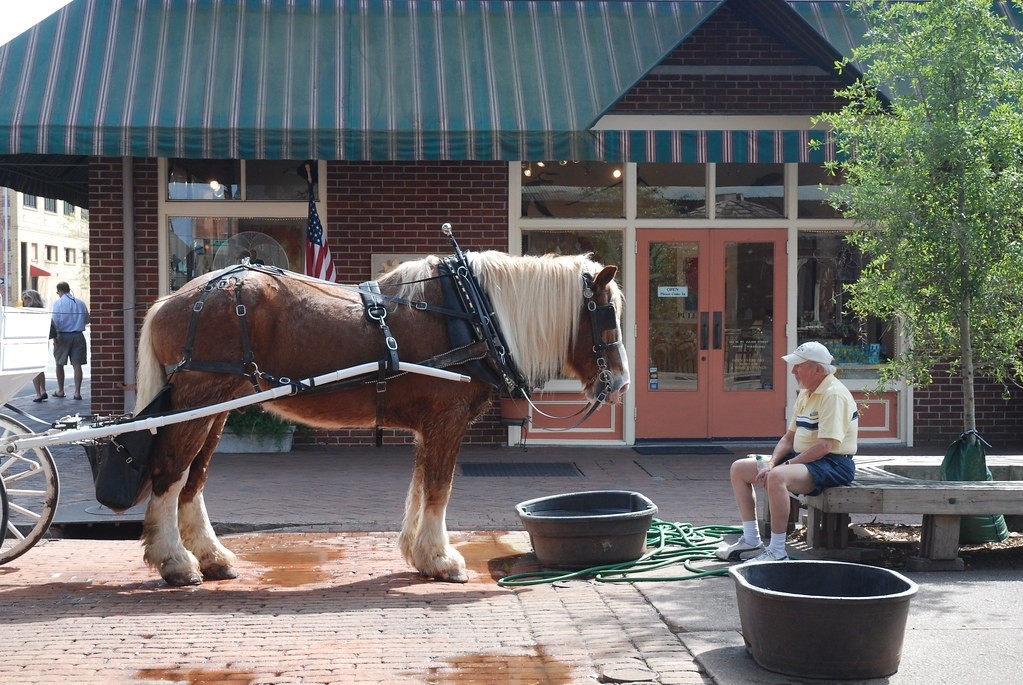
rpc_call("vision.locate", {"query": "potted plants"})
[213,403,312,456]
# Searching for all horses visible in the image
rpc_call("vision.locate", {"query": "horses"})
[133,250,632,587]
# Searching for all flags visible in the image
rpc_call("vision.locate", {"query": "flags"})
[306,197,337,281]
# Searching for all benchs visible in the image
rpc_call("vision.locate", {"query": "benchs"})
[746,453,1023,572]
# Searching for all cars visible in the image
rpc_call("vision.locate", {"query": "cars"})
[742,328,764,341]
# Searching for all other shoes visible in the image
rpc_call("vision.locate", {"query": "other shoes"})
[33,397,43,402]
[41,394,48,399]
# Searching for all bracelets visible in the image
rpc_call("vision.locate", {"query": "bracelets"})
[784,461,790,464]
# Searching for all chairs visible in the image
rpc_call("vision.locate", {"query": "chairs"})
[649,325,763,373]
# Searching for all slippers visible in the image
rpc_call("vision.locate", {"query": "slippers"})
[52,391,66,397]
[74,395,82,400]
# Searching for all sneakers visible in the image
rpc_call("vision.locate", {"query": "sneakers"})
[715,537,766,560]
[743,546,789,563]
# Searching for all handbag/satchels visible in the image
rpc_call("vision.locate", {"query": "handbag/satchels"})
[49,319,57,339]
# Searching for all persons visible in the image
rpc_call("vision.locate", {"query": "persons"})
[715,341,859,565]
[53,281,90,399]
[22,289,48,402]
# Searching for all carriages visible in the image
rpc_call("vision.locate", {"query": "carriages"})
[0,222,633,587]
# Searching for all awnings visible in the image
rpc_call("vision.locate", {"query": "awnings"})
[30,265,51,276]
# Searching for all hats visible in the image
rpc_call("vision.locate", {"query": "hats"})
[781,341,833,365]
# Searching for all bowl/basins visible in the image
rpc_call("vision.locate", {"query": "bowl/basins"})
[728,560,919,681]
[514,489,659,569]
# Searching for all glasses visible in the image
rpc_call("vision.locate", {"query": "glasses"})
[56,290,60,294]
[24,297,30,300]
